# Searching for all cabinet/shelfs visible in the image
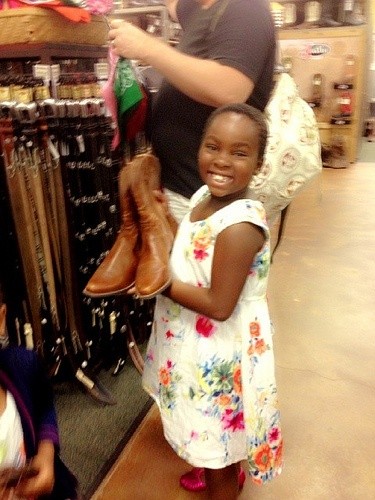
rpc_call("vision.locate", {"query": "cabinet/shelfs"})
[264,26,372,163]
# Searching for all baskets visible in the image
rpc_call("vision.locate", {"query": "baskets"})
[0,1,114,47]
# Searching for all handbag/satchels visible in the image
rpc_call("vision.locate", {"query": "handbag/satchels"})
[206,1,324,215]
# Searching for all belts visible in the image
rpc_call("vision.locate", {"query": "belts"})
[0,100,175,405]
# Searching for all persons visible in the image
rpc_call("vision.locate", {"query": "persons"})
[131,103,284,500]
[104,0,276,225]
[0,300,79,500]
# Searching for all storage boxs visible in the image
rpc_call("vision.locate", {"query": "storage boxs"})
[2,7,111,44]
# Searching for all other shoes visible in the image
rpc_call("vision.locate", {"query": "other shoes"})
[179,465,245,493]
